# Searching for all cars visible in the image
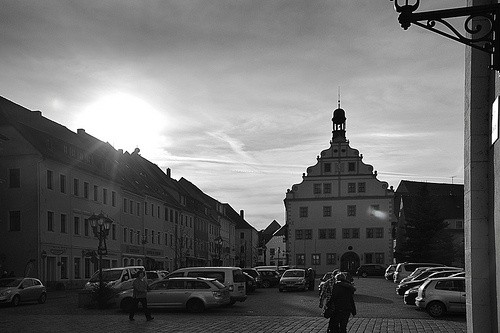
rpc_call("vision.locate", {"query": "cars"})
[242,266,291,289]
[116,277,231,313]
[146,270,170,285]
[414,277,466,317]
[0,277,47,306]
[385,264,398,280]
[397,266,466,304]
[280,269,309,291]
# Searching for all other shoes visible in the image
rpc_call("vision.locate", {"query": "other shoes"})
[129,318,134,321]
[147,317,154,321]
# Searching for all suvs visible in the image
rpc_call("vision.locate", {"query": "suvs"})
[356,264,387,278]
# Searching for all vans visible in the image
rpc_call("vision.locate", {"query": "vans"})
[165,266,247,303]
[82,266,147,293]
[393,263,448,283]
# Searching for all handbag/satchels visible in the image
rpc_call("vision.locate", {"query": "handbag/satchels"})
[323,306,334,319]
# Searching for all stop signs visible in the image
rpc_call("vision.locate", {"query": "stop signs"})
[41,251,47,258]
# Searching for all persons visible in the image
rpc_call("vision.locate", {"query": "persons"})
[308,266,315,291]
[350,261,360,279]
[128,269,155,321]
[318,267,356,333]
[362,272,368,278]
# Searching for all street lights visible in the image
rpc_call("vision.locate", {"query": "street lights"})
[89,209,113,285]
[215,234,224,265]
